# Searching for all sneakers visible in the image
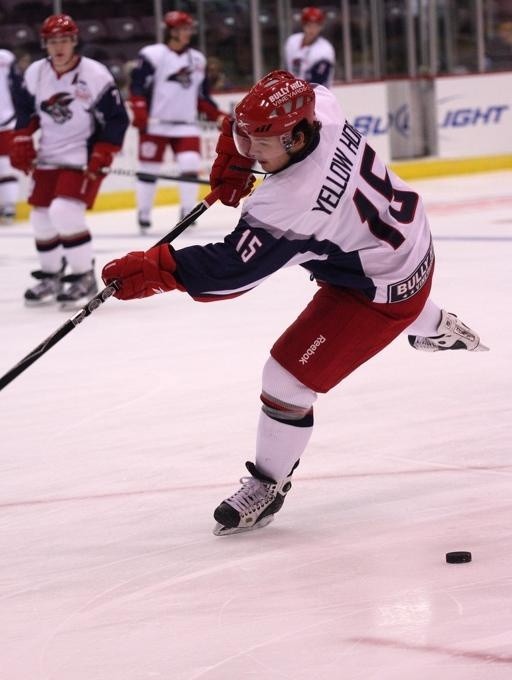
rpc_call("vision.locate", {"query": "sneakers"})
[139,210,151,226]
[408,308,481,353]
[213,457,293,527]
[181,209,197,224]
[24,265,99,304]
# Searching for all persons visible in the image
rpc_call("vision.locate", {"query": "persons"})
[282,6,334,90]
[11,14,129,308]
[0,50,25,219]
[101,68,488,535]
[127,9,228,232]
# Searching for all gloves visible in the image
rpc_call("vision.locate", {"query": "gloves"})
[130,99,148,131]
[201,104,228,131]
[206,138,255,206]
[85,144,115,176]
[9,136,39,174]
[100,242,178,300]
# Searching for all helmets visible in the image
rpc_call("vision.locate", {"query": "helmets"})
[165,12,197,32]
[39,15,80,40]
[302,7,325,27]
[235,71,315,140]
